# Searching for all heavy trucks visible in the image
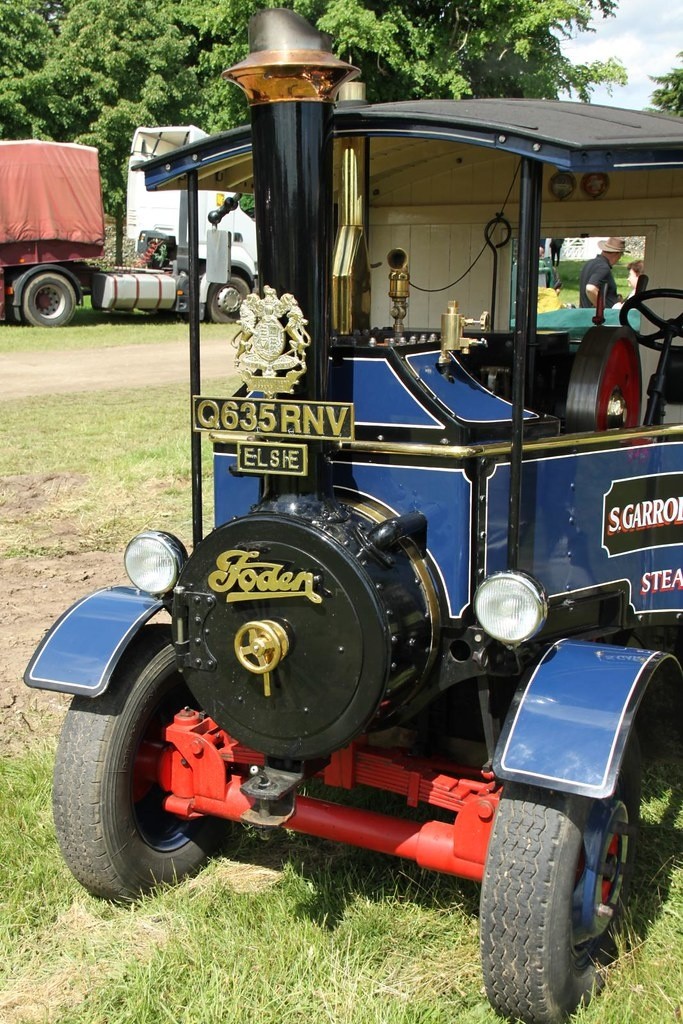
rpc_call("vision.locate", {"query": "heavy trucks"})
[0,125,258,327]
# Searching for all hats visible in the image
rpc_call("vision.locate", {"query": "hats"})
[598,238,632,254]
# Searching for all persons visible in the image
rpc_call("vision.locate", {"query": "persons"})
[550,238,564,267]
[612,260,644,309]
[579,237,631,310]
[539,247,562,296]
